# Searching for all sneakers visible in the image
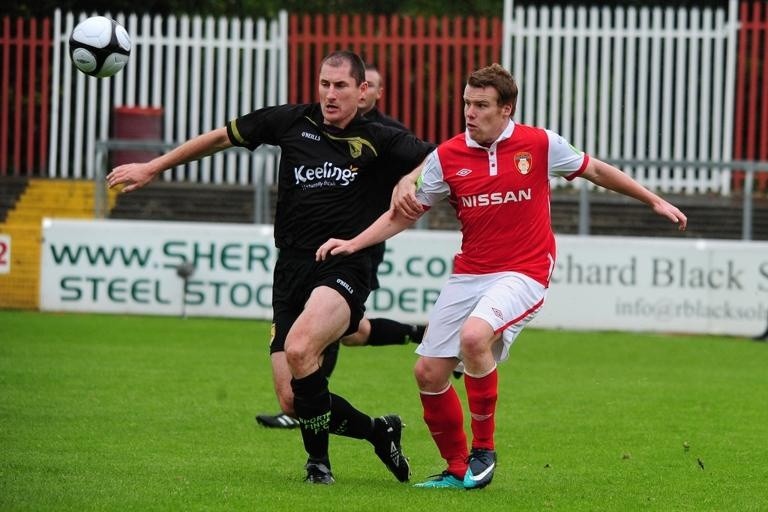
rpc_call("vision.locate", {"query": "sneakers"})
[304,458,335,484]
[374,415,412,483]
[256,413,300,429]
[413,449,497,489]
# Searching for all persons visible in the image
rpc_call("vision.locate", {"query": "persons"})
[316,63,688,489]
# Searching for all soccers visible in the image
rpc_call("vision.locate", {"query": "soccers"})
[69,16,131,77]
